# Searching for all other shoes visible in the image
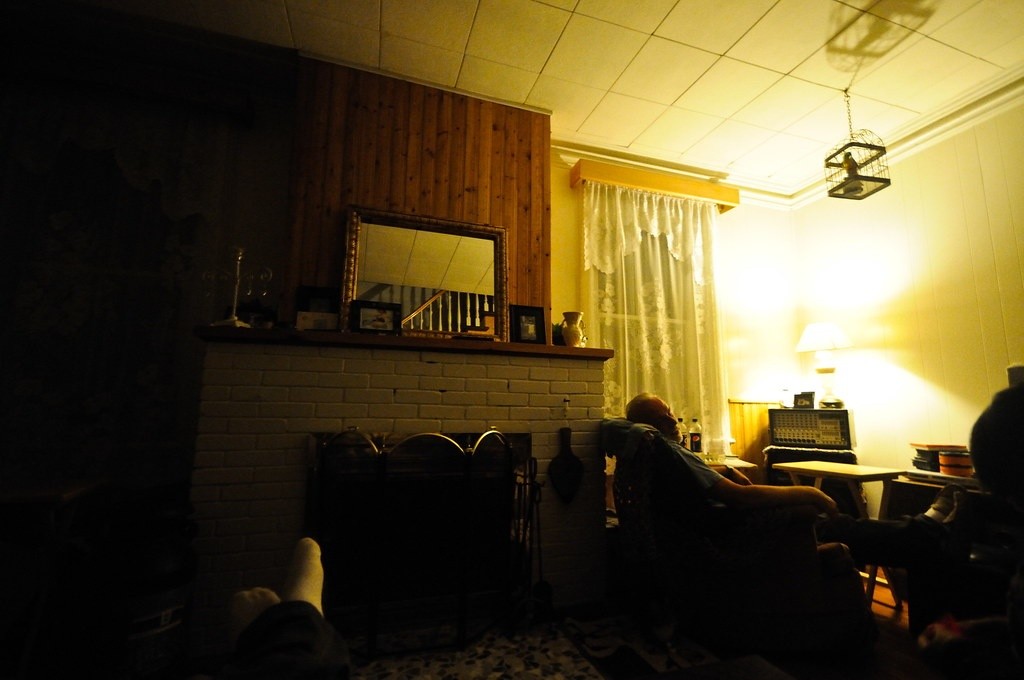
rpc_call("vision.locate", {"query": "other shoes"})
[930,484,972,562]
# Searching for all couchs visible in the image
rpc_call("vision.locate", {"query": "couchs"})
[910,382,1024,680]
[599,419,880,664]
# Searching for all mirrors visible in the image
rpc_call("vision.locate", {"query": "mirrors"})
[338,206,509,342]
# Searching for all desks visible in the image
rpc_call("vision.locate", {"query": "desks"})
[892,479,987,505]
[771,460,909,616]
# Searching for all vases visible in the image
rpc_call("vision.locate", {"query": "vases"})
[561,311,585,346]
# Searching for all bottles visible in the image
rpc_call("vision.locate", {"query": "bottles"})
[676,418,687,450]
[689,418,704,460]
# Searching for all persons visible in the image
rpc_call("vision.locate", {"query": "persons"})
[626,393,967,564]
[226,537,352,680]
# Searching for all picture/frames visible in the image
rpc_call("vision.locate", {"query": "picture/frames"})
[349,300,402,333]
[509,304,547,345]
[295,285,339,314]
